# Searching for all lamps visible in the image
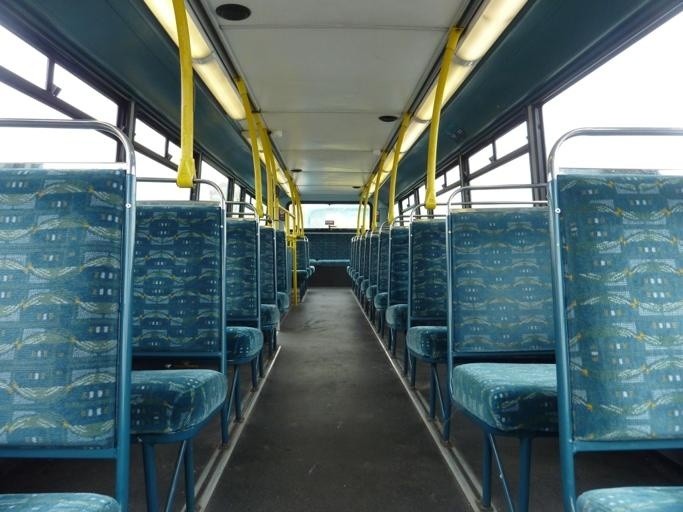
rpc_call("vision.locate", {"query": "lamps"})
[362,0,537,199]
[140,0,291,198]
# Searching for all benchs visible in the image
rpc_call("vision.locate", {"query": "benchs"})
[304,232,357,286]
